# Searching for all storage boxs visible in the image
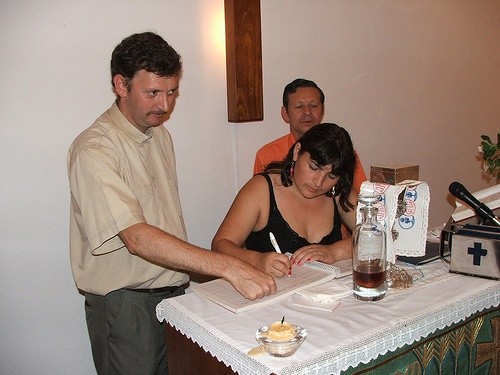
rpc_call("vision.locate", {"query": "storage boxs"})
[368,163,419,185]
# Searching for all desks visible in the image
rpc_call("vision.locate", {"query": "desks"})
[155,225,500,375]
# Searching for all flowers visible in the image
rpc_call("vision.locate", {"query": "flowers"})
[477,133,500,183]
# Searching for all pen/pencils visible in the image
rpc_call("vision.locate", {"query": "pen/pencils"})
[269,231,291,279]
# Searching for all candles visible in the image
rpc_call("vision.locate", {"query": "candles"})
[269,316,292,340]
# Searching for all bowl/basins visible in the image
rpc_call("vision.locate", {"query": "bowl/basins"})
[256,323,306,357]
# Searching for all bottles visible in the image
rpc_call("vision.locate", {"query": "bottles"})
[351,195,387,301]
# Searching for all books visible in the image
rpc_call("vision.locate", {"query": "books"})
[190,251,351,315]
[397,241,451,266]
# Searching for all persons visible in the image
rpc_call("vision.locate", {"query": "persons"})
[66,32,278,375]
[214,122,362,278]
[252,78,368,239]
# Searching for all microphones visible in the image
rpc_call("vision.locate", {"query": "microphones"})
[448,181,500,227]
